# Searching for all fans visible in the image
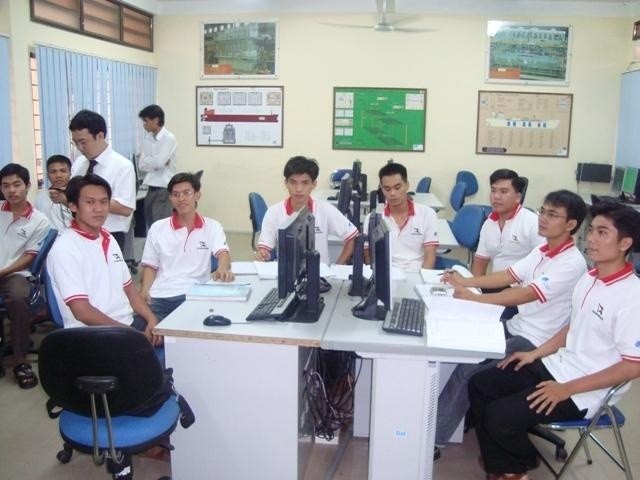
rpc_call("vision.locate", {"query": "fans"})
[318,0,436,34]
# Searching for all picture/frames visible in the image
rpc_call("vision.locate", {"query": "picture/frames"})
[484,20,573,88]
[332,89,427,152]
[476,90,573,158]
[196,86,284,147]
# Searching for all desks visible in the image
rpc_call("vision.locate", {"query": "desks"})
[321,266,505,480]
[152,261,343,480]
[325,189,444,214]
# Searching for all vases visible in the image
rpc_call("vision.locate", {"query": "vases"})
[198,19,280,80]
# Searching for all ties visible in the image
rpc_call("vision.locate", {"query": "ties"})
[86,160,97,176]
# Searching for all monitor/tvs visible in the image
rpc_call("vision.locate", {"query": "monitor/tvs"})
[274,211,324,322]
[352,160,367,201]
[348,208,383,296]
[350,218,390,320]
[338,172,361,232]
[370,182,385,212]
[297,205,332,293]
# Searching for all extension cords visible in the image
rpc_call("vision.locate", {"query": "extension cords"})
[314,414,342,444]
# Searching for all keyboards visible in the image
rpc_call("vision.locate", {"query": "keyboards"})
[336,191,353,200]
[382,296,425,336]
[246,287,299,320]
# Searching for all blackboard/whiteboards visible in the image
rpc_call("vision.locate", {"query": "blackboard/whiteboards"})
[613,68,640,168]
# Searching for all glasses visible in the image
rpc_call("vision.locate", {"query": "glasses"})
[537,209,567,218]
[171,191,193,197]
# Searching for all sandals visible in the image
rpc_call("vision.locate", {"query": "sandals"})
[489,472,529,480]
[13,364,38,388]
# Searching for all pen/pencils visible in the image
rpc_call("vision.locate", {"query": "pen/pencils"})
[438,270,458,276]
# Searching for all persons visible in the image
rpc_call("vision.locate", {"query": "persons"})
[1,163,51,390]
[253,156,360,264]
[435,190,589,462]
[49,109,136,253]
[34,155,76,228]
[141,173,234,322]
[364,163,439,268]
[135,104,177,236]
[45,174,164,371]
[471,169,545,276]
[468,201,640,480]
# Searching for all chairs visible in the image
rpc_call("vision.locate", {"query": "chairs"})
[28,229,57,305]
[41,271,63,326]
[249,192,276,261]
[536,380,635,480]
[412,164,536,269]
[330,168,352,189]
[37,327,180,480]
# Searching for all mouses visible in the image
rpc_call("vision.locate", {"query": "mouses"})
[327,196,337,200]
[203,314,231,326]
[408,191,415,196]
